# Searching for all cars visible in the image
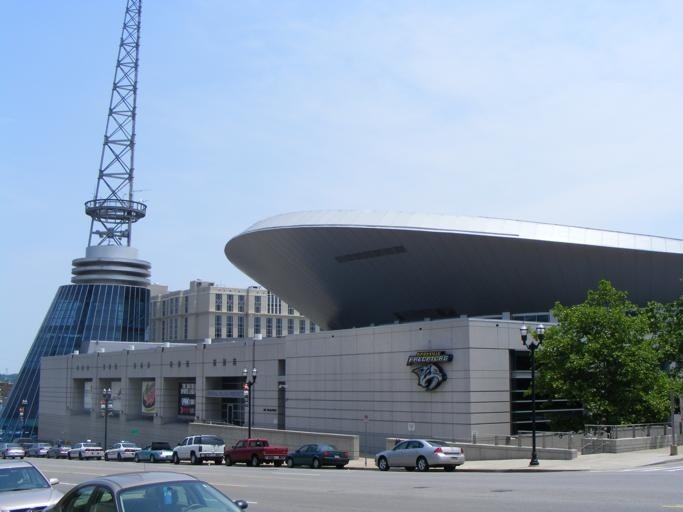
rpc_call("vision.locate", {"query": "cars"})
[284,443,349,469]
[374,438,464,472]
[0,437,174,463]
[0,458,65,511]
[41,471,247,511]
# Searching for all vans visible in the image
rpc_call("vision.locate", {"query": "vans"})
[172,434,226,465]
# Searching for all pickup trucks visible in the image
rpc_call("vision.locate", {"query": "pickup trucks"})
[224,437,287,467]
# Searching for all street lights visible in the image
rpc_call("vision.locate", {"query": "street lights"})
[519,322,545,466]
[666,360,679,454]
[21,399,27,439]
[102,387,111,450]
[242,367,257,438]
